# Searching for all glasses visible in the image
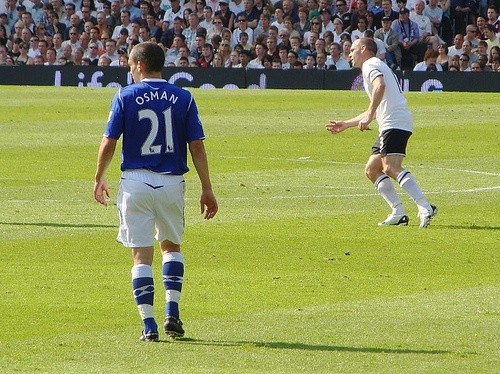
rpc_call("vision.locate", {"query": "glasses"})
[23,26,108,50]
[336,4,344,7]
[219,4,227,6]
[312,22,319,26]
[82,11,89,13]
[459,59,467,62]
[469,31,477,33]
[65,9,72,11]
[214,22,222,24]
[17,9,23,11]
[103,7,110,9]
[472,67,479,69]
[118,52,125,55]
[238,19,246,22]
[196,2,203,5]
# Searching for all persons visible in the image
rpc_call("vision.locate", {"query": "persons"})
[0,0,500,72]
[93,43,219,341]
[326,38,439,229]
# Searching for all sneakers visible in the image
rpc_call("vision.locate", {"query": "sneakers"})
[419,204,438,228]
[378,214,409,226]
[163,317,185,336]
[139,330,158,342]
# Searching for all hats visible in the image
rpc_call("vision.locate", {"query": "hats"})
[320,8,330,15]
[17,5,26,10]
[398,8,409,14]
[382,16,390,21]
[117,48,127,54]
[120,28,128,34]
[219,0,228,4]
